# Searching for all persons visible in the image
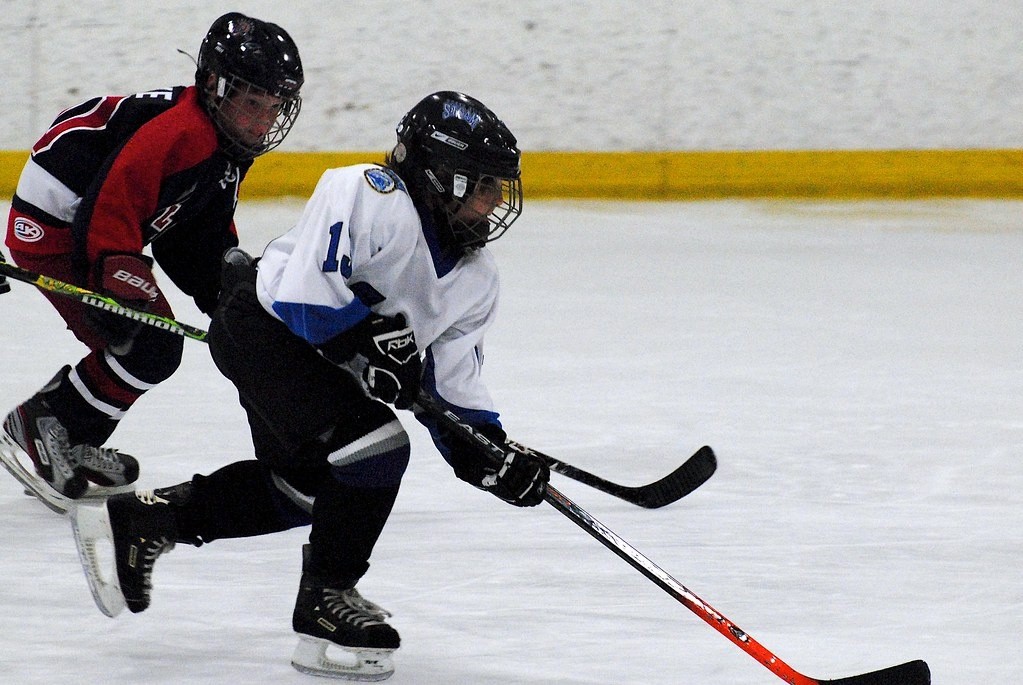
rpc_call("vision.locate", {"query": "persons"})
[0,12,305,513]
[74,92,549,682]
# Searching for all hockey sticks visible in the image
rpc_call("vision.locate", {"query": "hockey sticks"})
[1,261,212,344]
[403,391,932,685]
[505,441,719,512]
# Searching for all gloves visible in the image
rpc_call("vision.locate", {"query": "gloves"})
[451,427,550,508]
[350,312,423,409]
[87,247,173,347]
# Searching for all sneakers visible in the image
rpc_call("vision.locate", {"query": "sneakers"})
[71,479,192,617]
[23,444,141,497]
[0,365,89,515]
[292,546,400,681]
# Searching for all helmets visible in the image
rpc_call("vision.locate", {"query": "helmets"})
[194,12,305,158]
[392,92,523,252]
